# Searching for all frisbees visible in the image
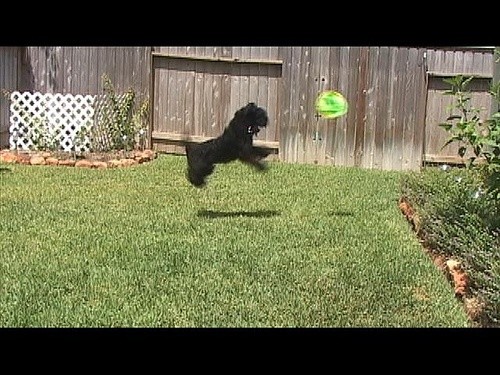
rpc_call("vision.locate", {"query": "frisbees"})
[314,88,349,120]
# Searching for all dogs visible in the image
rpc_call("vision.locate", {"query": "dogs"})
[182,103,272,186]
